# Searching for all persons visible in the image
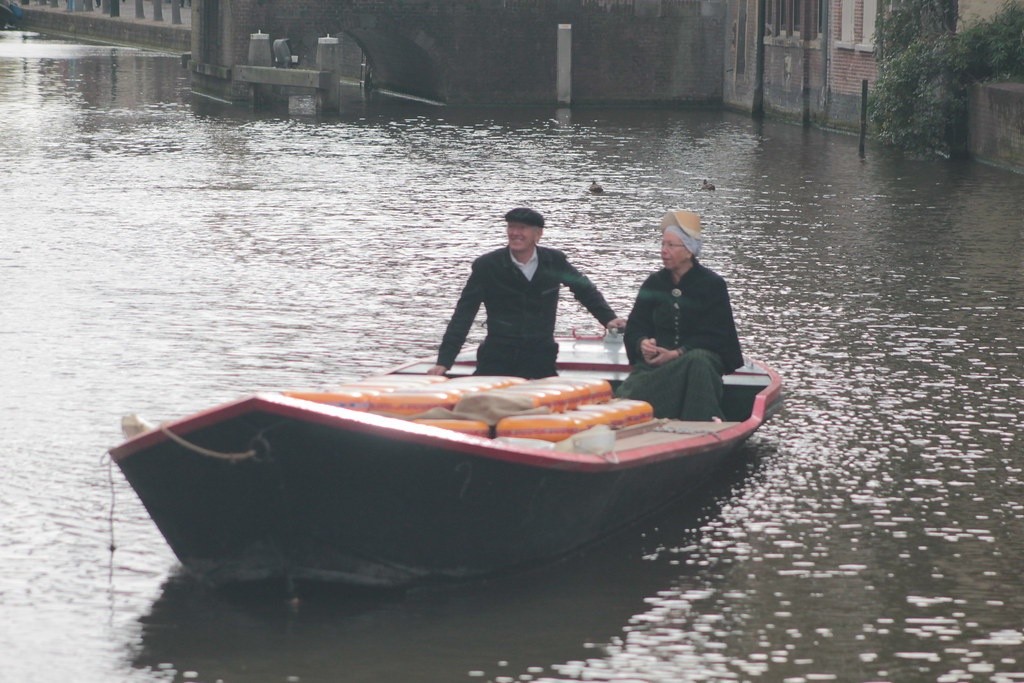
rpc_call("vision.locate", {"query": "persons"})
[428,207,627,384]
[612,212,743,425]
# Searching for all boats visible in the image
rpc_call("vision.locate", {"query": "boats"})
[106,322,784,591]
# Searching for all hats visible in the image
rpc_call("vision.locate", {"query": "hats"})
[660,211,700,239]
[503,207,544,228]
[664,225,700,257]
